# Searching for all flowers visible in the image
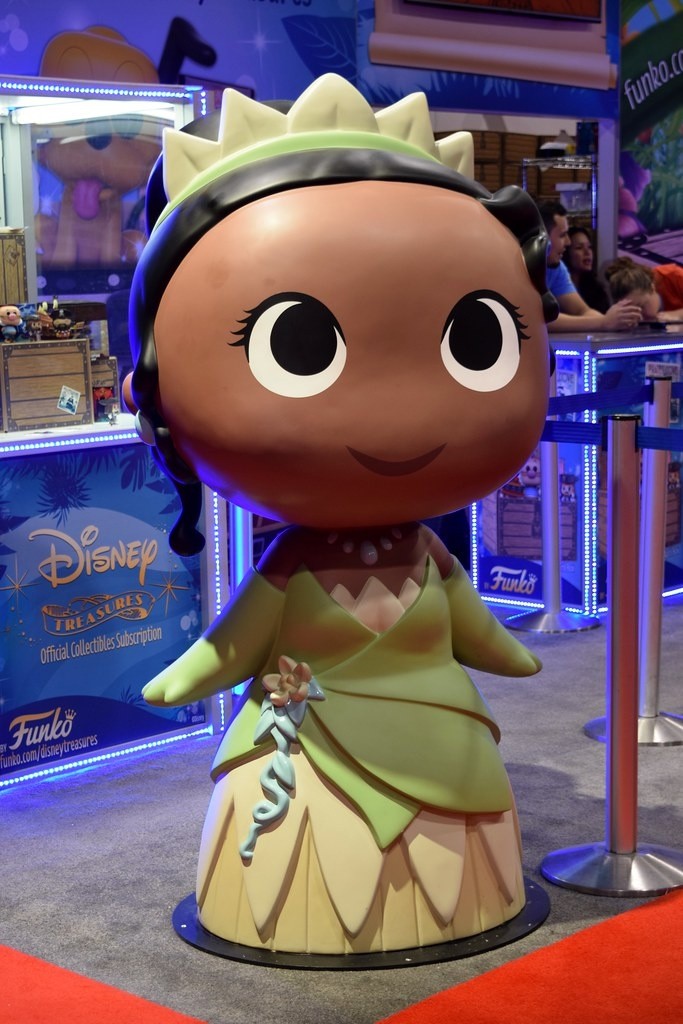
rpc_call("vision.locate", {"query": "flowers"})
[261,654,311,707]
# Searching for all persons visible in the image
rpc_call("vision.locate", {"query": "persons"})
[0,305,73,343]
[121,69,565,955]
[99,397,119,427]
[536,199,683,332]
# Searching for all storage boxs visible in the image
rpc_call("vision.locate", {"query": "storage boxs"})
[0,335,94,434]
